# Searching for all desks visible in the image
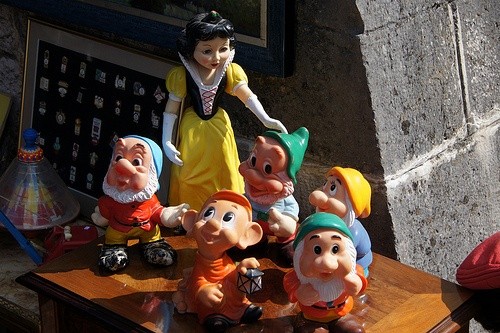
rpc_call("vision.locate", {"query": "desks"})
[15,223,492,333]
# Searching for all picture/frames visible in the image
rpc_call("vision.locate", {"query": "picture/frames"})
[18,0,294,222]
